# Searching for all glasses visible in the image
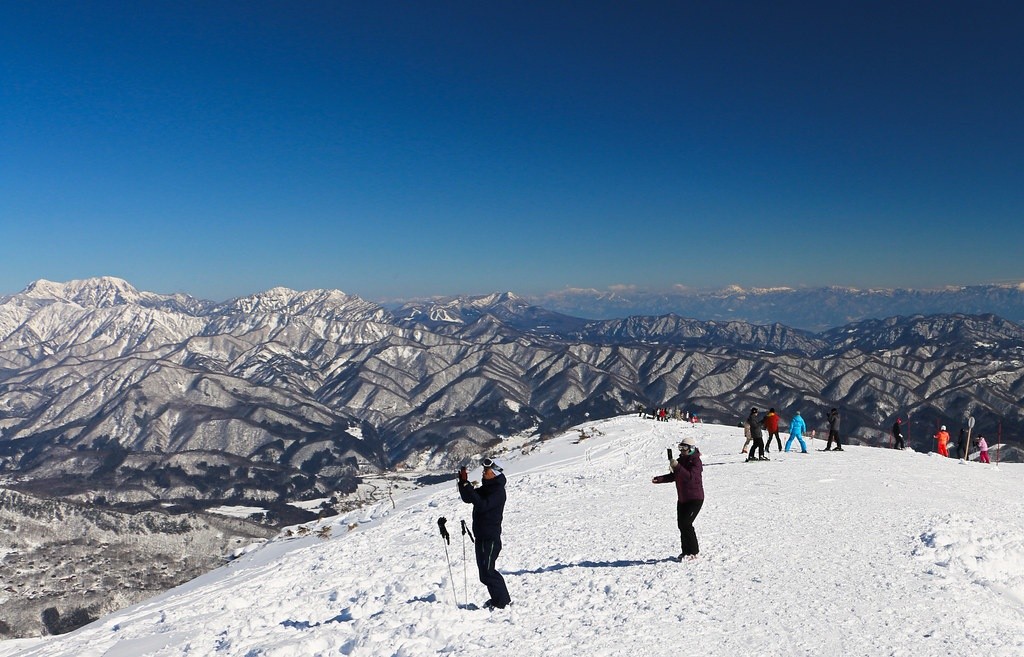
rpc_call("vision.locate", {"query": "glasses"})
[481,458,503,472]
[678,444,689,451]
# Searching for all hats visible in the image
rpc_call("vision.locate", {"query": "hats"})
[681,438,696,455]
[751,407,758,413]
[489,460,503,476]
[941,425,946,431]
[831,408,836,413]
[897,418,901,424]
[770,407,775,413]
[960,428,965,431]
[796,411,801,415]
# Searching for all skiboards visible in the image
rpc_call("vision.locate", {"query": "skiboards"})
[743,458,786,461]
[815,449,844,451]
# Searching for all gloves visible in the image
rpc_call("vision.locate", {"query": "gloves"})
[670,459,680,468]
[458,466,467,480]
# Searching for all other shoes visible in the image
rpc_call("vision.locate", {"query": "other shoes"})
[801,450,807,453]
[985,462,990,464]
[833,447,843,451]
[742,447,782,461]
[824,447,831,451]
[483,599,493,609]
[489,603,505,612]
[894,447,901,449]
[979,460,984,463]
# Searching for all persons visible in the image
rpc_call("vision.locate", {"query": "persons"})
[892,418,905,450]
[639,404,703,428]
[741,407,843,461]
[651,437,705,560]
[933,425,948,457]
[956,428,970,461]
[976,434,989,464]
[458,459,511,608]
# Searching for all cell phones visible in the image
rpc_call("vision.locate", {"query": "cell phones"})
[666,448,673,461]
[460,465,466,480]
